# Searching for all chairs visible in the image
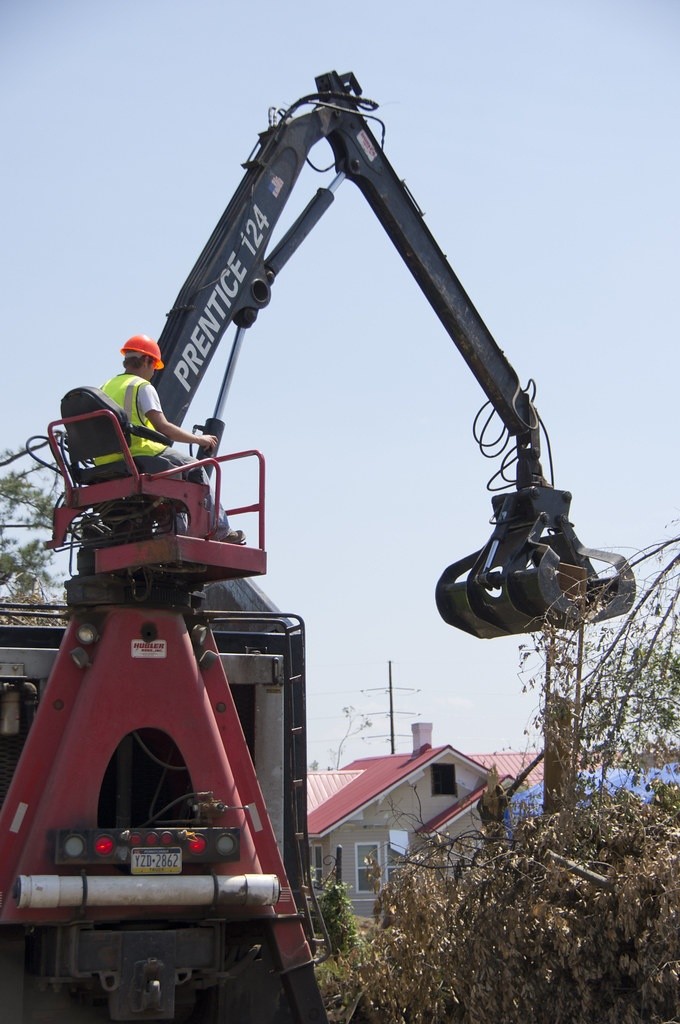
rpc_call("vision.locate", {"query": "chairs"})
[60,385,183,480]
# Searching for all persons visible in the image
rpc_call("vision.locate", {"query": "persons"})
[91,334,245,546]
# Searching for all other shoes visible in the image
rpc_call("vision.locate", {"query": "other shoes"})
[220,530,246,543]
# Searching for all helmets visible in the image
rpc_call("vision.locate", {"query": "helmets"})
[120,334,164,370]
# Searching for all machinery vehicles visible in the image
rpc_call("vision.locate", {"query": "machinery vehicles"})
[1,68,638,1024]
